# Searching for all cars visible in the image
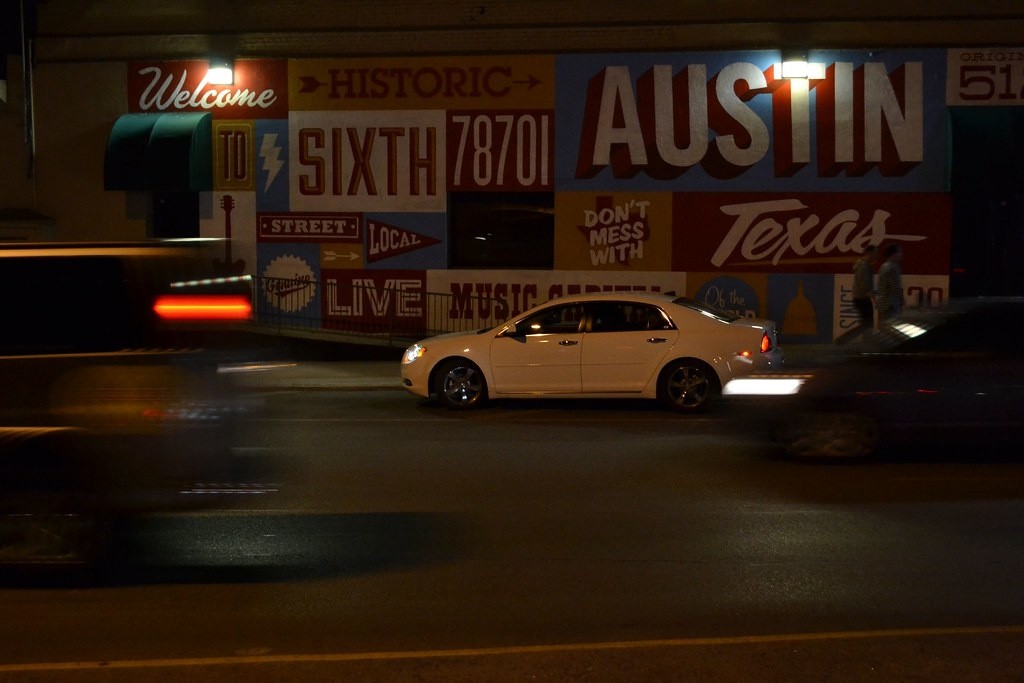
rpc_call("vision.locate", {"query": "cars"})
[400,291,782,416]
[744,295,1024,467]
